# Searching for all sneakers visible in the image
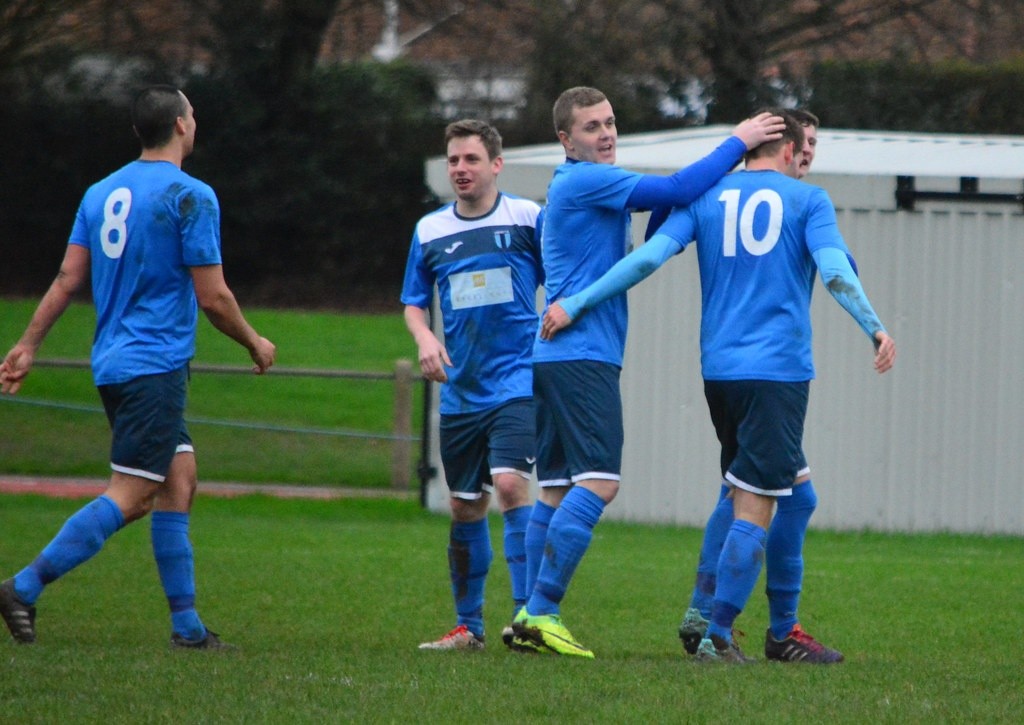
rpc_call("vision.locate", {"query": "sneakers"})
[512,636,555,655]
[0,578,36,642]
[511,604,595,658]
[171,626,236,650]
[418,625,484,650]
[678,608,710,654]
[502,625,514,645]
[697,639,758,666]
[766,624,843,665]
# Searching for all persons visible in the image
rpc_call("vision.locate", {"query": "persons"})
[0,85,276,650]
[399,120,543,650]
[719,109,845,662]
[540,107,897,662]
[509,86,786,660]
